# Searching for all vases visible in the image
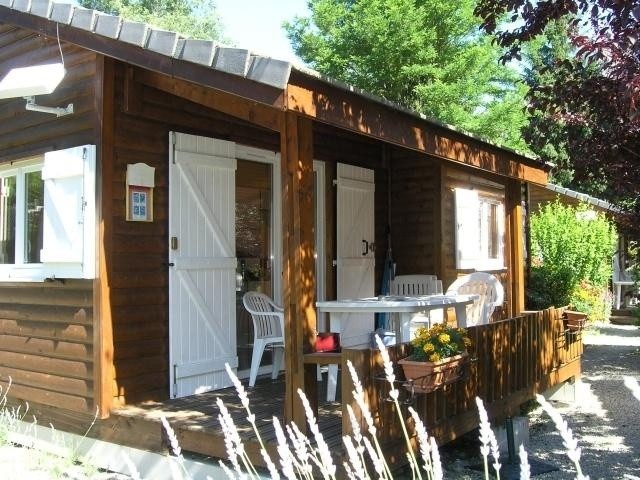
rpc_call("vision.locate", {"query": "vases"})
[397,351,471,394]
[564,310,588,334]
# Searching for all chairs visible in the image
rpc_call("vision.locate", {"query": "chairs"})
[439,272,504,329]
[372,272,444,337]
[242,291,324,388]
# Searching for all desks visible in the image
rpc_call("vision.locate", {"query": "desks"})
[614,281,640,310]
[313,292,479,403]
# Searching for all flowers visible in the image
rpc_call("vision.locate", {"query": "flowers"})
[408,319,471,363]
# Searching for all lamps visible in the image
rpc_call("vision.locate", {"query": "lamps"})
[1,63,76,119]
